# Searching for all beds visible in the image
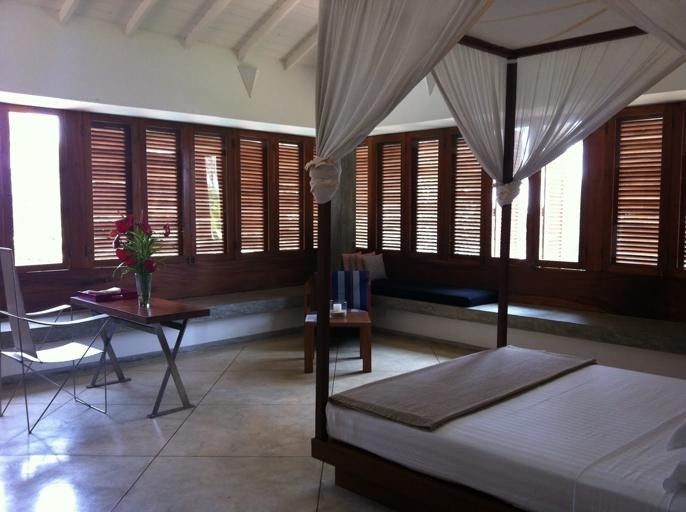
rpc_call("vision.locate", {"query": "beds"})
[326,344,685,512]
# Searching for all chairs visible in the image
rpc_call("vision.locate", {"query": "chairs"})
[0,247,110,434]
[304,270,372,374]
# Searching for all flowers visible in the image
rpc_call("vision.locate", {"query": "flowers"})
[109,213,170,273]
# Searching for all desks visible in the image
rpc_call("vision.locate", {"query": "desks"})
[69,291,210,419]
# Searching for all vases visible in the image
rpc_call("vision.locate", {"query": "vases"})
[135,271,151,310]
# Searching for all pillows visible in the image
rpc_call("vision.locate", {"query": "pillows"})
[342,251,387,280]
